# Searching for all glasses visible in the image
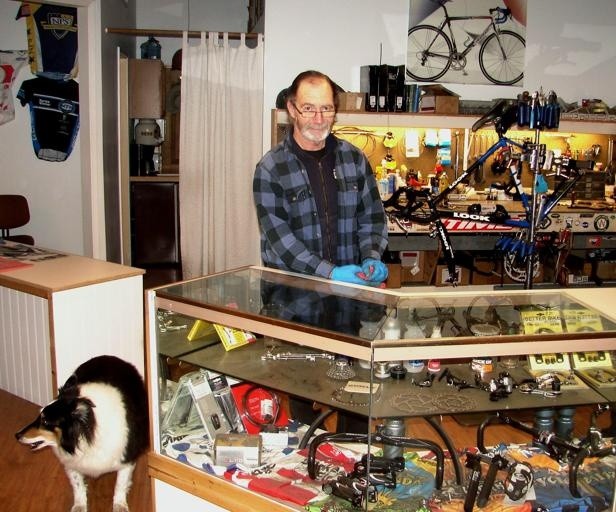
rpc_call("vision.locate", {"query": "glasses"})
[293,104,335,118]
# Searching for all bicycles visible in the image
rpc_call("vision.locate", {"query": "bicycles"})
[406,1,526,86]
[381,100,585,286]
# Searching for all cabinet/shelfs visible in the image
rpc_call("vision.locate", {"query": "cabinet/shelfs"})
[270,109,614,254]
[146,264,615,512]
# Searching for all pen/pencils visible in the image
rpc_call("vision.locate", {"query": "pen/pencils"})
[438,367,449,381]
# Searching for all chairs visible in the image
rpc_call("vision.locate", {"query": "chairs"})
[0,194,35,245]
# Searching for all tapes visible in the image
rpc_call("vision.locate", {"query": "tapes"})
[402,360,425,373]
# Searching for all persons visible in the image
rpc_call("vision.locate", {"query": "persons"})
[252,70,389,433]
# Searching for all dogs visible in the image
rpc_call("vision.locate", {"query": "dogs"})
[15,355,149,512]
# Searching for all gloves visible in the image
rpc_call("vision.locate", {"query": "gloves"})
[330,258,388,286]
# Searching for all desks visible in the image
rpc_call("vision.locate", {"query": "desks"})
[0,242,145,407]
